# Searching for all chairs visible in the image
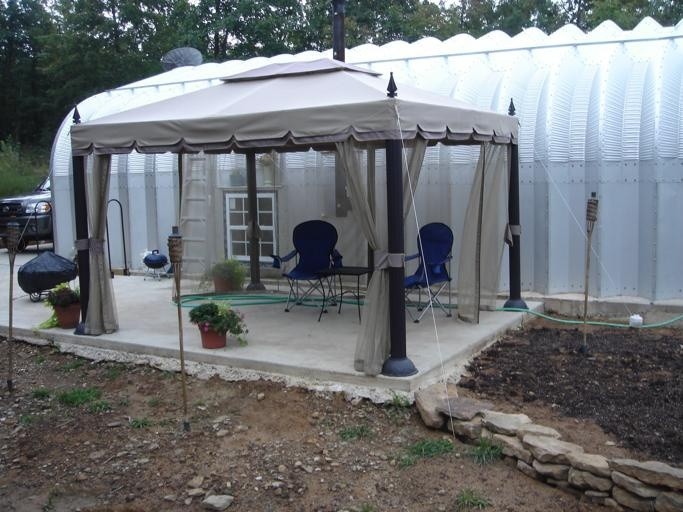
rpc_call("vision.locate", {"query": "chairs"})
[268,220,343,313]
[404,222,454,323]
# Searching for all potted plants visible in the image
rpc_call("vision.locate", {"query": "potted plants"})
[197,257,247,291]
[188,301,249,349]
[39,283,80,329]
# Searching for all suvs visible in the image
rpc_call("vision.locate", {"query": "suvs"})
[0,175,52,253]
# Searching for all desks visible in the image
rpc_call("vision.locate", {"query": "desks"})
[314,266,373,324]
